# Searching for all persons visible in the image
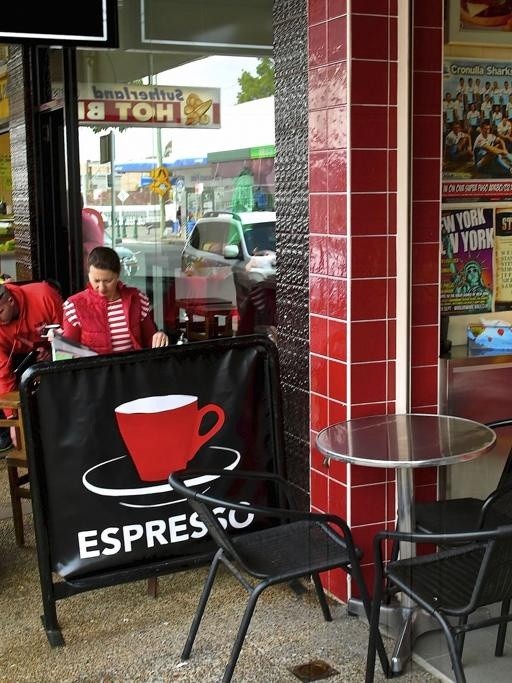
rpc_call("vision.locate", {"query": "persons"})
[61,246,170,355]
[252,225,278,256]
[443,77,512,176]
[82,195,105,287]
[0,281,65,452]
[172,206,195,234]
[228,157,278,251]
[446,241,494,310]
[203,226,224,255]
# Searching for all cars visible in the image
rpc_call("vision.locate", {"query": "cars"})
[103,230,139,276]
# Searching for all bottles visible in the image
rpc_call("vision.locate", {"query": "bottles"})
[0,201,6,214]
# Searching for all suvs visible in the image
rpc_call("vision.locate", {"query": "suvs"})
[182,210,276,309]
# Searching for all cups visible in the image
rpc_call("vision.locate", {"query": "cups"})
[44,324,61,334]
[115,394,225,482]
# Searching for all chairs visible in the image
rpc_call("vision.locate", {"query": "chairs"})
[167,466,396,682]
[359,450,512,682]
[0,391,32,546]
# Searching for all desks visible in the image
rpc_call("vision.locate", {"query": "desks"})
[188,305,237,338]
[316,414,497,673]
[174,297,231,330]
[15,331,51,347]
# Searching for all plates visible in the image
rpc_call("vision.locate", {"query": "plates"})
[81,446,240,508]
